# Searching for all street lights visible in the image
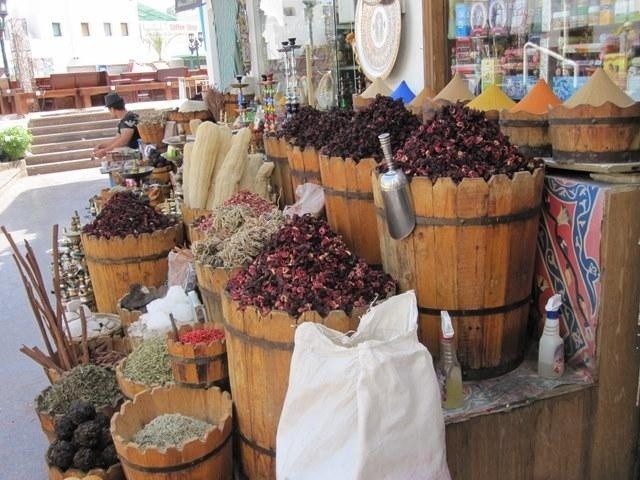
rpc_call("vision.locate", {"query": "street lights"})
[302,0,318,45]
[0,0,13,90]
[189,32,204,71]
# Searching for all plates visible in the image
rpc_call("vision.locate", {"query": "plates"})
[352,1,402,81]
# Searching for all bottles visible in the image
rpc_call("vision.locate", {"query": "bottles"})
[569,1,601,27]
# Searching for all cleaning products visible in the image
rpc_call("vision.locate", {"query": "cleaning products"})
[537,294,564,379]
[435,310,464,410]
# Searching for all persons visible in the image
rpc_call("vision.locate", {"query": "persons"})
[91,90,143,161]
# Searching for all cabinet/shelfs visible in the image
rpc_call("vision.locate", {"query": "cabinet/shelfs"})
[443,0,640,104]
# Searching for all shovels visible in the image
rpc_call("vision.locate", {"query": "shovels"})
[377,131,417,242]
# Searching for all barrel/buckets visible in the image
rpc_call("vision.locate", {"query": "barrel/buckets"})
[350,94,639,168]
[33,364,117,444]
[110,384,233,480]
[224,213,396,480]
[117,283,153,325]
[44,411,123,480]
[169,324,229,388]
[79,191,185,310]
[193,191,282,323]
[42,335,143,384]
[373,165,543,378]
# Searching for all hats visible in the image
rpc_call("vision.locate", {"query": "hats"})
[104,92,123,107]
